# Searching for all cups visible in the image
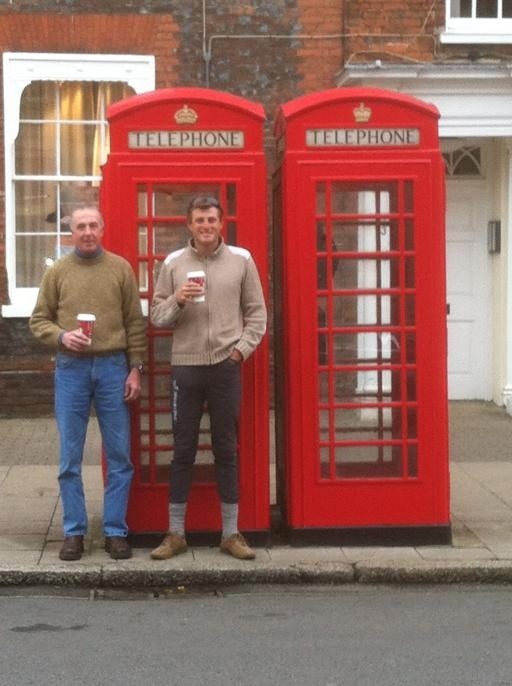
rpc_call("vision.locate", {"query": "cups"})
[187,271,206,302]
[77,314,96,346]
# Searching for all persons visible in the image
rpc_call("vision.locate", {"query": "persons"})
[29,205,148,558]
[150,192,269,563]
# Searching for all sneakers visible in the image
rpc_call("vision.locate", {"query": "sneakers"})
[150,532,189,562]
[104,535,134,561]
[220,530,257,561]
[58,536,86,562]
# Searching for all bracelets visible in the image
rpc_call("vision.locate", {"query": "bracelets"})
[131,362,146,372]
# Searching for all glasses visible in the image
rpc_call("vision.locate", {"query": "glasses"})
[192,196,220,209]
